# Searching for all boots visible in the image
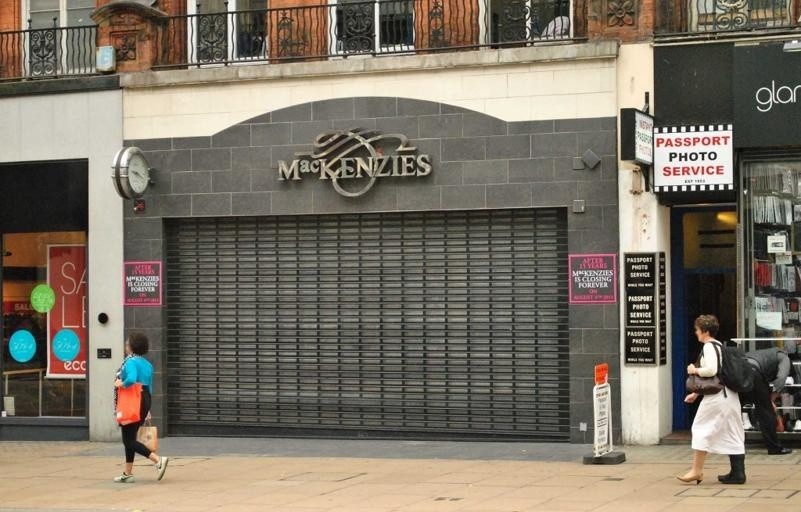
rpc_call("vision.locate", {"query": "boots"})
[717,455,746,483]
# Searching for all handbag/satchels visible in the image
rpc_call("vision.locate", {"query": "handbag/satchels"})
[685,375,723,395]
[136,418,157,452]
[115,382,141,425]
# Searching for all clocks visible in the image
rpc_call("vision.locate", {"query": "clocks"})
[110,147,151,200]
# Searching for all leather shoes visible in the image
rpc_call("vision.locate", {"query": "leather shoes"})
[768,447,792,455]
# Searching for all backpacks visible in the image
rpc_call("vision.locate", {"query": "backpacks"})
[702,341,753,397]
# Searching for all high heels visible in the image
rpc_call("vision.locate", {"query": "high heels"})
[677,473,704,484]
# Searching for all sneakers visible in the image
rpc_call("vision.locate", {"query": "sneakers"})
[114,472,135,483]
[155,457,168,481]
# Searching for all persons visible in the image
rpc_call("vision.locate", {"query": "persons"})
[737,345,795,455]
[677,313,748,484]
[114,332,169,483]
